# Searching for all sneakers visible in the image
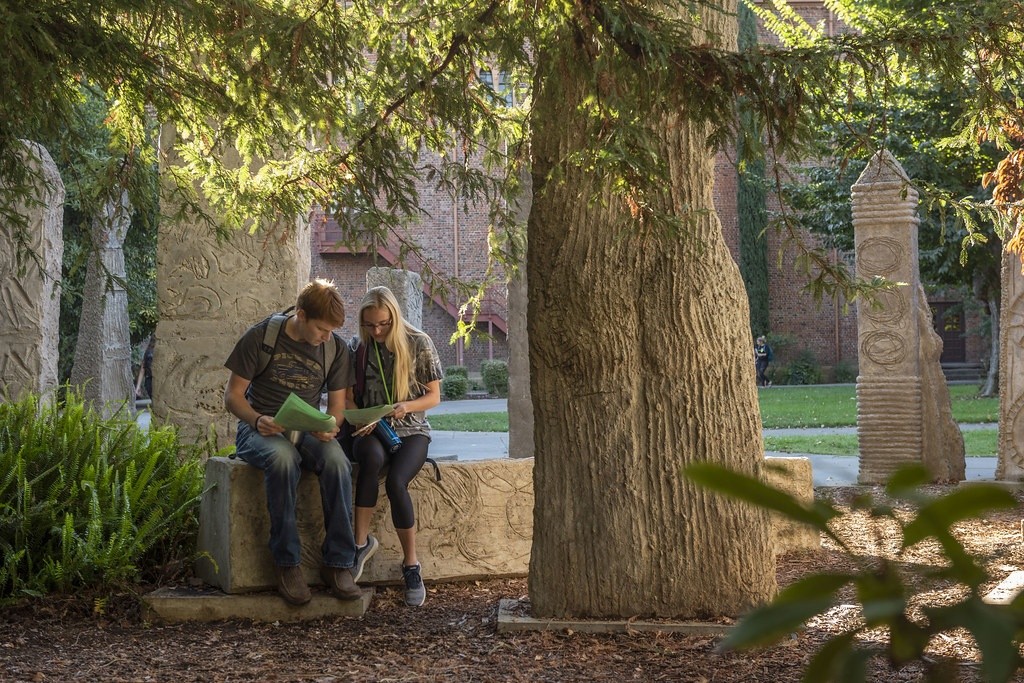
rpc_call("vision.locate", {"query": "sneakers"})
[400,559,426,607]
[353,534,379,582]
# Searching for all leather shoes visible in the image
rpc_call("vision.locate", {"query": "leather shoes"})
[320,565,361,601]
[275,564,312,605]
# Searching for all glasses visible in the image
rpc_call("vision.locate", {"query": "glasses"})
[360,318,393,330]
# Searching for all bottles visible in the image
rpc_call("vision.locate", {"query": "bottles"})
[374,417,403,453]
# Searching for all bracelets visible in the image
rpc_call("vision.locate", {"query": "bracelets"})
[255,414,263,432]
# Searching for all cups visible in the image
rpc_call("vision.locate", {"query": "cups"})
[284,428,302,447]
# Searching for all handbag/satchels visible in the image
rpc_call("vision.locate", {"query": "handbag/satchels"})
[335,398,364,463]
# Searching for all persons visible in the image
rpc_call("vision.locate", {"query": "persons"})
[136,331,155,400]
[224,278,444,607]
[753,335,772,388]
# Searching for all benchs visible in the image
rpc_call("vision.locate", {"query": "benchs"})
[196,455,821,592]
